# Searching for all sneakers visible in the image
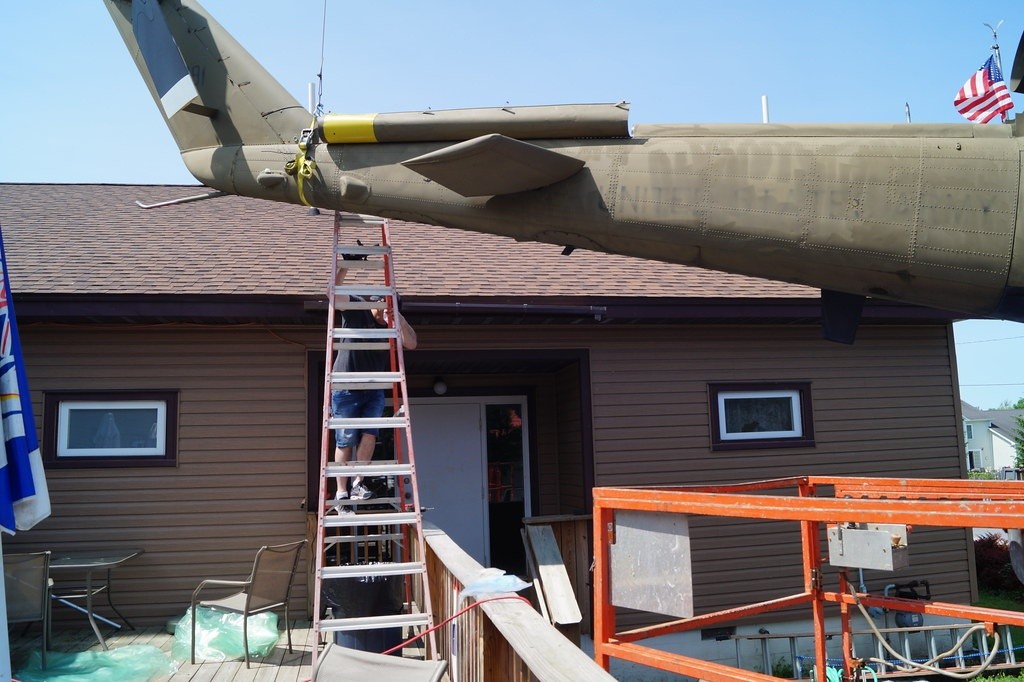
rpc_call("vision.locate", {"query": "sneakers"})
[350,484,377,500]
[332,496,356,516]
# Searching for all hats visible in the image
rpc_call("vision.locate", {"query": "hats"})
[370,291,403,312]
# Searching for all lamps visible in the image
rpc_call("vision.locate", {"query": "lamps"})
[433,378,447,395]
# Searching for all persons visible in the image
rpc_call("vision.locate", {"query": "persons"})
[324,240,417,516]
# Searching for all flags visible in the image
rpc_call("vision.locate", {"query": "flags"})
[954,54,1013,124]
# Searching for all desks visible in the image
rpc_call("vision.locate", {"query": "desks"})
[48,547,144,651]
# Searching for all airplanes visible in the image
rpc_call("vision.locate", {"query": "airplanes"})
[104,0,1024,330]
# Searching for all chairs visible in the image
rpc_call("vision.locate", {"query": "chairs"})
[191,539,308,667]
[2,551,51,672]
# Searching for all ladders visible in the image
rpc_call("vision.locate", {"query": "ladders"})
[312,211,438,672]
